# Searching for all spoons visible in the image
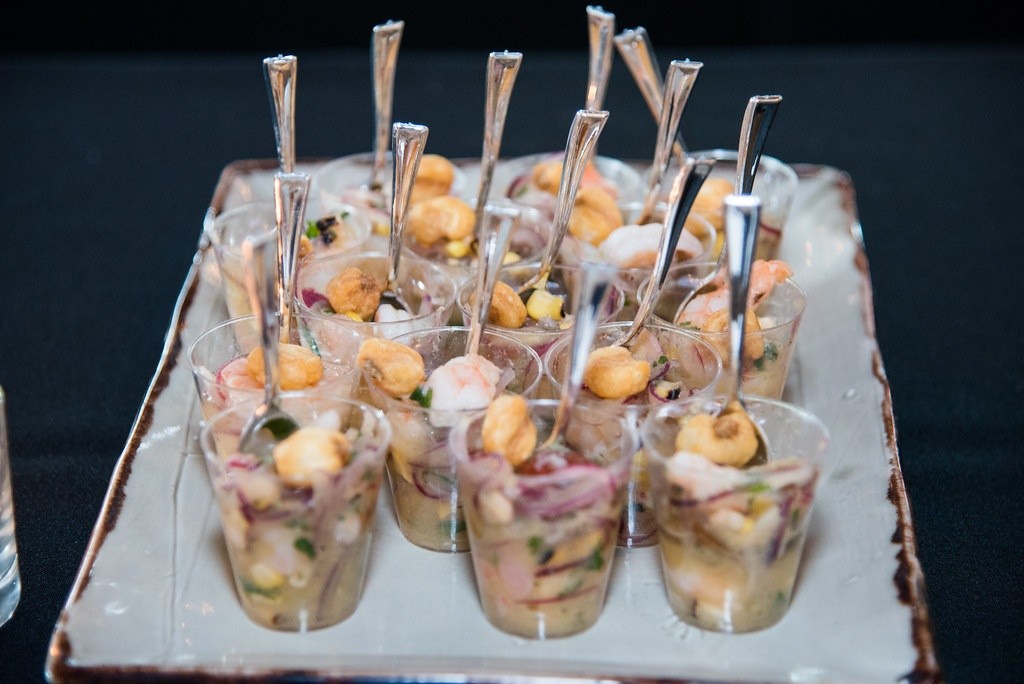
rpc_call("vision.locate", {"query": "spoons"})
[235,7,774,470]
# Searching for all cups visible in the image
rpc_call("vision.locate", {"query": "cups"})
[205,148,810,402]
[187,313,395,634]
[446,398,641,641]
[641,390,831,636]
[361,325,722,554]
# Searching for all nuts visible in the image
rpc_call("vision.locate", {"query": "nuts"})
[251,153,757,483]
